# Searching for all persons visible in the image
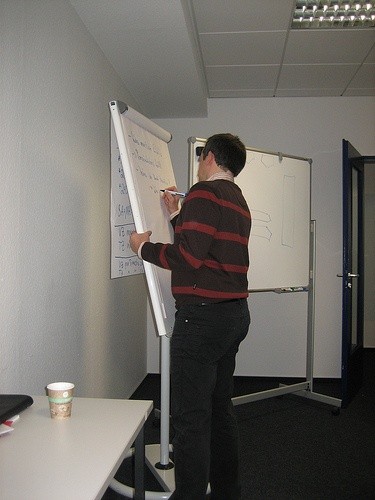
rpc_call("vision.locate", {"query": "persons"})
[128,132,253,499]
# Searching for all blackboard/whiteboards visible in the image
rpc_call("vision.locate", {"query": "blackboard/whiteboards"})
[189,136,312,293]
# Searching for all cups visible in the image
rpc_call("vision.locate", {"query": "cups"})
[47,382,75,420]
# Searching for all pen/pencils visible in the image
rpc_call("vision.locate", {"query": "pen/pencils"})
[161,189,188,198]
[278,287,308,292]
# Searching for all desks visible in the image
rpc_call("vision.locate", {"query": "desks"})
[0,396,153,500]
